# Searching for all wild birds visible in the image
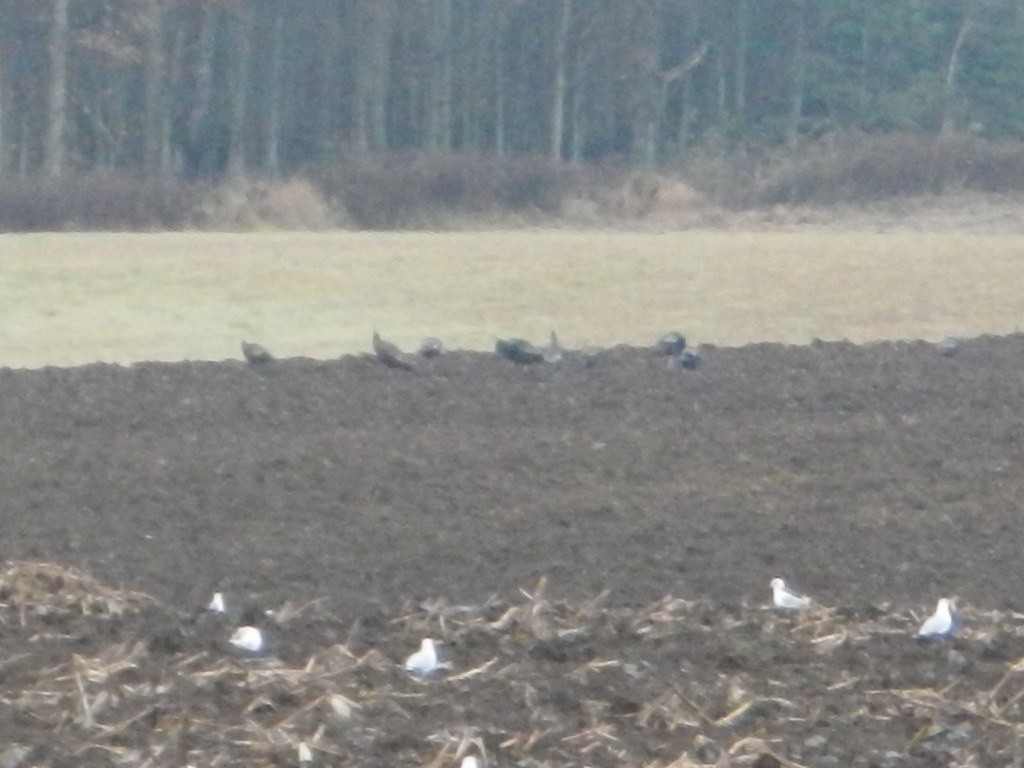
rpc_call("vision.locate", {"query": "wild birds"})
[210,593,266,654]
[912,598,953,642]
[239,328,706,377]
[406,638,445,675]
[769,577,812,616]
